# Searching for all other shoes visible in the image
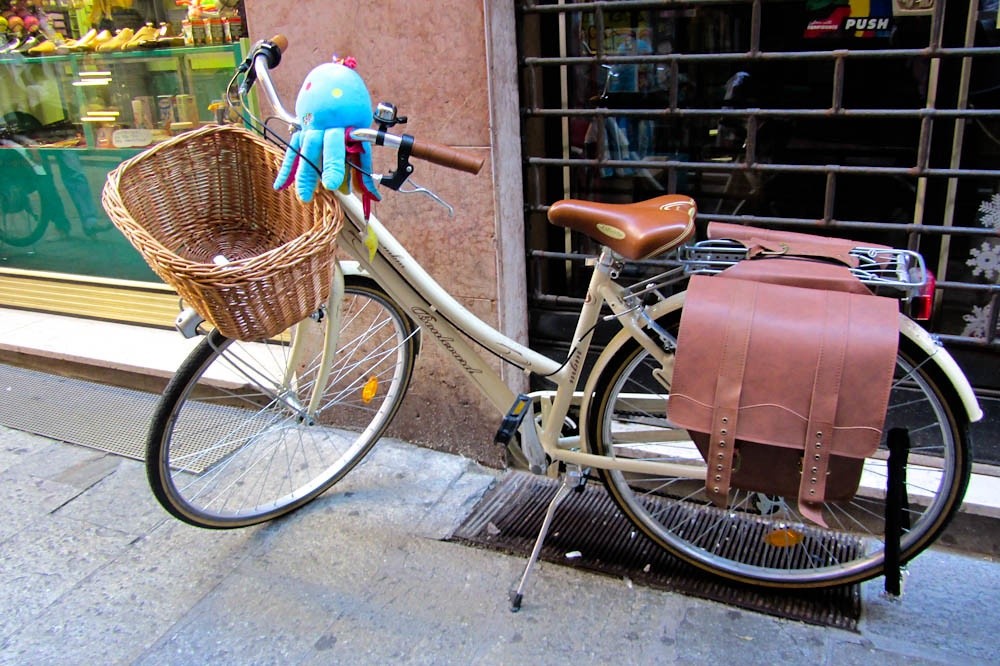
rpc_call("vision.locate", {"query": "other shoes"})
[86,220,114,237]
[0,28,157,56]
[45,230,68,243]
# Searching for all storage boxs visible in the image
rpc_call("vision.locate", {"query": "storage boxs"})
[134,93,201,133]
[181,15,241,47]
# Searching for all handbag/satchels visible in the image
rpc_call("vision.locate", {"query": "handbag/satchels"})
[666,222,901,530]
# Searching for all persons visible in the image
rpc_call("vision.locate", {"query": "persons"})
[0,63,117,242]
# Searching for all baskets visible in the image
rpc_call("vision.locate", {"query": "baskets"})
[101,122,344,342]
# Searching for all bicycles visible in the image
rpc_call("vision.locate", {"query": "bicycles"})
[0,140,52,248]
[96,28,986,612]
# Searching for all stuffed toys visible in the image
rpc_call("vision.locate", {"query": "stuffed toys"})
[272,56,383,265]
